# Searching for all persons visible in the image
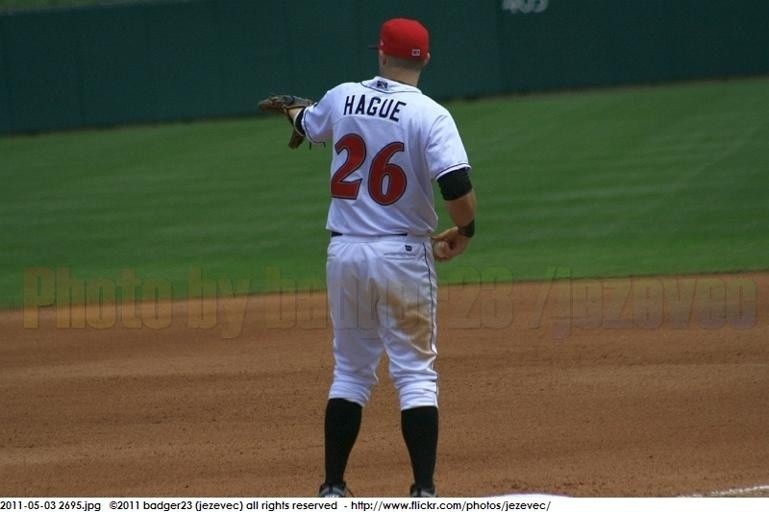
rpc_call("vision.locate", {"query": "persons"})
[257,17,477,497]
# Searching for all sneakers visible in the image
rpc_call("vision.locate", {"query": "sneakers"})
[410,485,437,497]
[318,482,349,497]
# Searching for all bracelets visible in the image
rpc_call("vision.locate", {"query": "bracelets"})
[458,219,474,238]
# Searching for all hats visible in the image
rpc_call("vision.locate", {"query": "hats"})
[369,18,430,59]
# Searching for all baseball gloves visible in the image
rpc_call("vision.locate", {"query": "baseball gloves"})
[257,94,315,149]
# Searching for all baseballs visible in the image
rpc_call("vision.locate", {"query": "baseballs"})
[429,240,450,260]
